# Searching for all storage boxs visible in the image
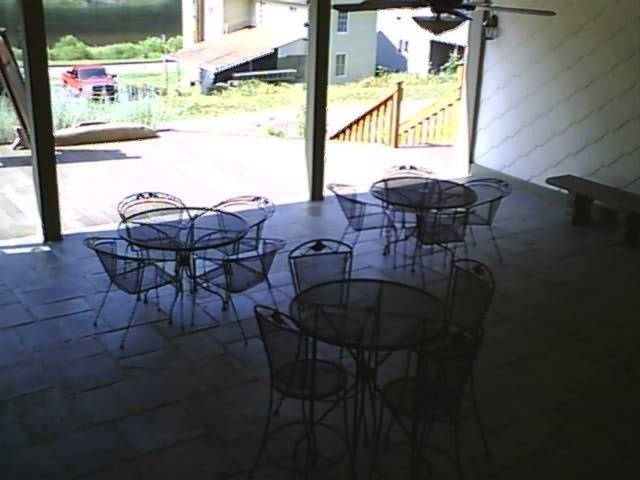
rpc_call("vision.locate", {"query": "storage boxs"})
[544,173,640,238]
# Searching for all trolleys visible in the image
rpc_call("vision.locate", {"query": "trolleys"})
[331,0,561,23]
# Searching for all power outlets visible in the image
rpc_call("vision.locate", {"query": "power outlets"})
[63,64,119,103]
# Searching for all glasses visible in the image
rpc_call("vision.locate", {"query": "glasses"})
[480,14,498,41]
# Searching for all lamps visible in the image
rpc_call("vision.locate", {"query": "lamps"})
[251,302,353,473]
[190,194,277,291]
[404,199,474,275]
[376,321,488,475]
[117,192,198,278]
[326,181,401,274]
[450,176,511,258]
[83,234,186,350]
[409,256,500,406]
[289,236,361,368]
[193,235,278,350]
